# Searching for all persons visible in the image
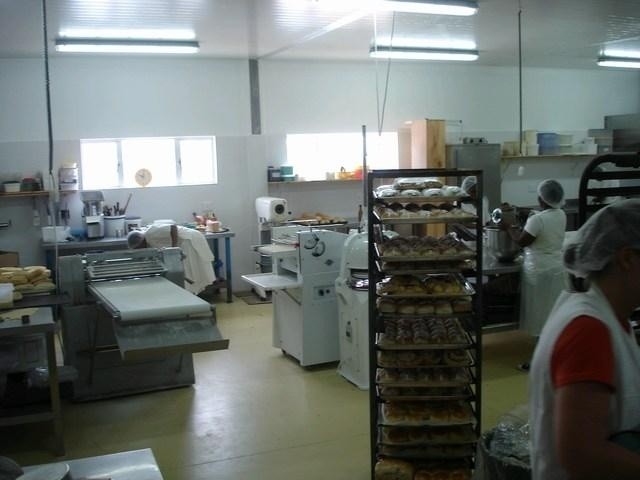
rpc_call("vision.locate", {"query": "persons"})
[497,179,569,374]
[458,175,492,251]
[126,218,219,296]
[527,197,640,479]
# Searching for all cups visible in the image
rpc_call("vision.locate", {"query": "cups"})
[0,283,13,309]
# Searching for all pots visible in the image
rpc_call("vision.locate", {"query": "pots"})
[483,226,524,264]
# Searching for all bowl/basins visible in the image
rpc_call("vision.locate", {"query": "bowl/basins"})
[40,225,71,245]
[3,180,21,193]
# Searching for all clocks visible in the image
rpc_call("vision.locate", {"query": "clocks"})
[134,168,152,186]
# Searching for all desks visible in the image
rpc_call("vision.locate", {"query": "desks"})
[2,308,63,455]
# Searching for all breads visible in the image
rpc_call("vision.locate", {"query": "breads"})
[293,211,347,225]
[370,177,475,479]
[0,264,57,295]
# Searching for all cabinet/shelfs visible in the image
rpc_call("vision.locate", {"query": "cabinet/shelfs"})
[362,169,487,476]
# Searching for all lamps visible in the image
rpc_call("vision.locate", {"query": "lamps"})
[596,50,640,70]
[369,45,478,62]
[53,34,199,55]
[378,1,482,18]
